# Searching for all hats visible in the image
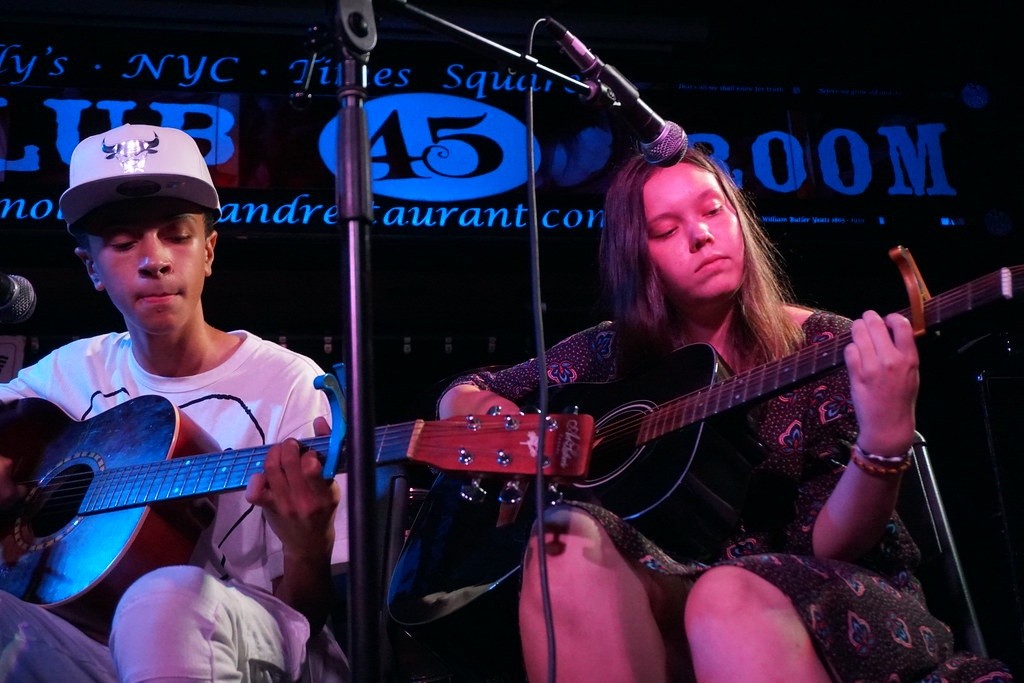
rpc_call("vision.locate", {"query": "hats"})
[59,123,221,237]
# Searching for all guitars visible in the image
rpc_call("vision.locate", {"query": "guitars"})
[0,393,598,642]
[386,237,1024,642]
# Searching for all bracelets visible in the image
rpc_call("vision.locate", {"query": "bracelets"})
[850,446,911,477]
[855,442,906,463]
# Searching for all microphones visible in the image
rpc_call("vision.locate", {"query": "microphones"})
[0,271,36,325]
[544,16,687,168]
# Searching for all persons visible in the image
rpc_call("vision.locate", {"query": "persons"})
[436,146,920,683]
[0,121,352,683]
